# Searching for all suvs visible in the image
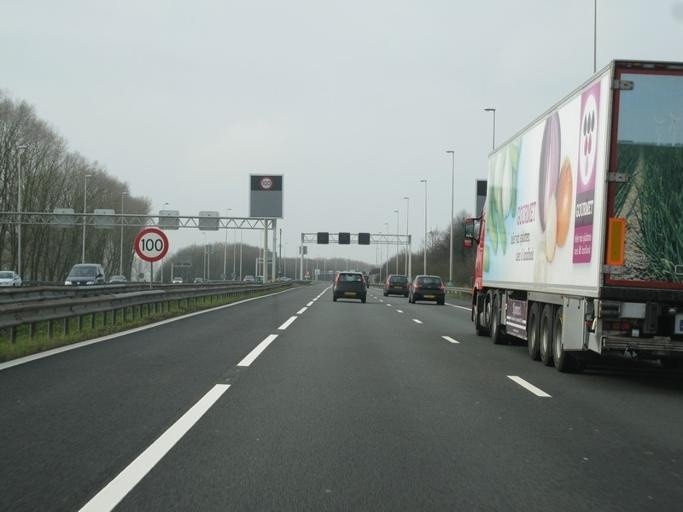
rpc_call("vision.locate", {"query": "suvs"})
[382,274,445,305]
[333,271,367,303]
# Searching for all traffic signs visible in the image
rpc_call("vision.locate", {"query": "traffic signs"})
[134,228,169,261]
[260,178,272,190]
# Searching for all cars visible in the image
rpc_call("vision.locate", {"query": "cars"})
[63,262,126,284]
[242,275,254,283]
[0,270,22,287]
[172,276,206,284]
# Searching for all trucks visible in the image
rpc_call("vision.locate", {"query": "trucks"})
[463,61,681,375]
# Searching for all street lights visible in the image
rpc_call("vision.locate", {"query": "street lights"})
[484,107,494,152]
[378,198,409,280]
[118,191,126,273]
[15,145,26,271]
[224,207,230,280]
[81,175,90,264]
[445,151,453,285]
[420,180,426,274]
